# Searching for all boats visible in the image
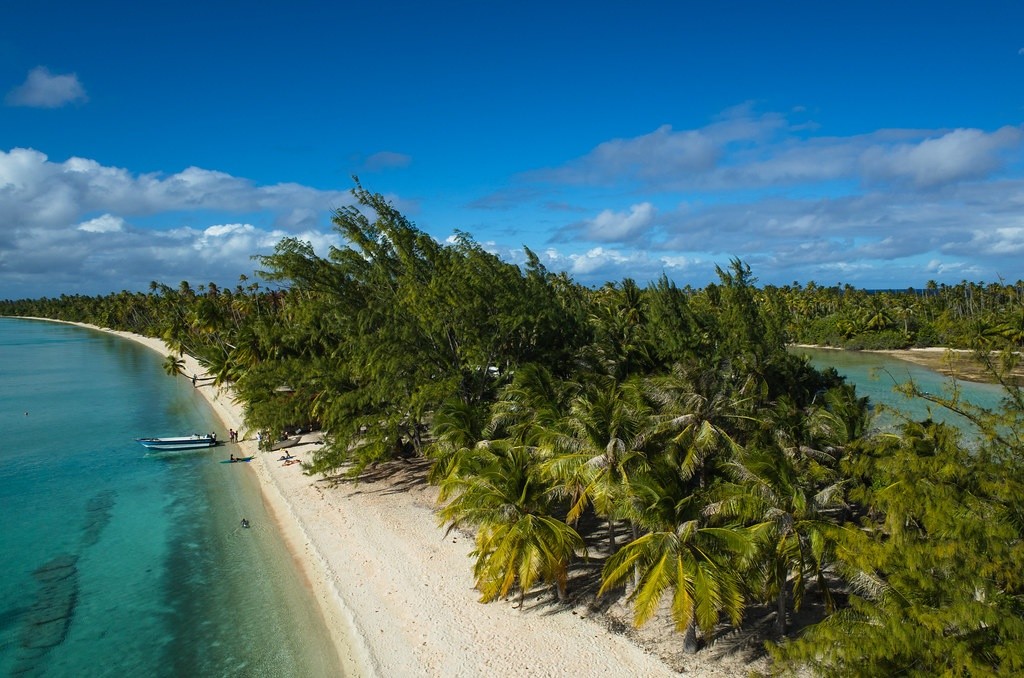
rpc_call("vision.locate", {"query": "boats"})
[134,434,216,450]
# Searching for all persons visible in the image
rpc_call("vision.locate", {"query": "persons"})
[230,454,241,462]
[282,459,302,466]
[211,432,217,444]
[229,429,289,446]
[191,373,198,388]
[276,450,290,462]
